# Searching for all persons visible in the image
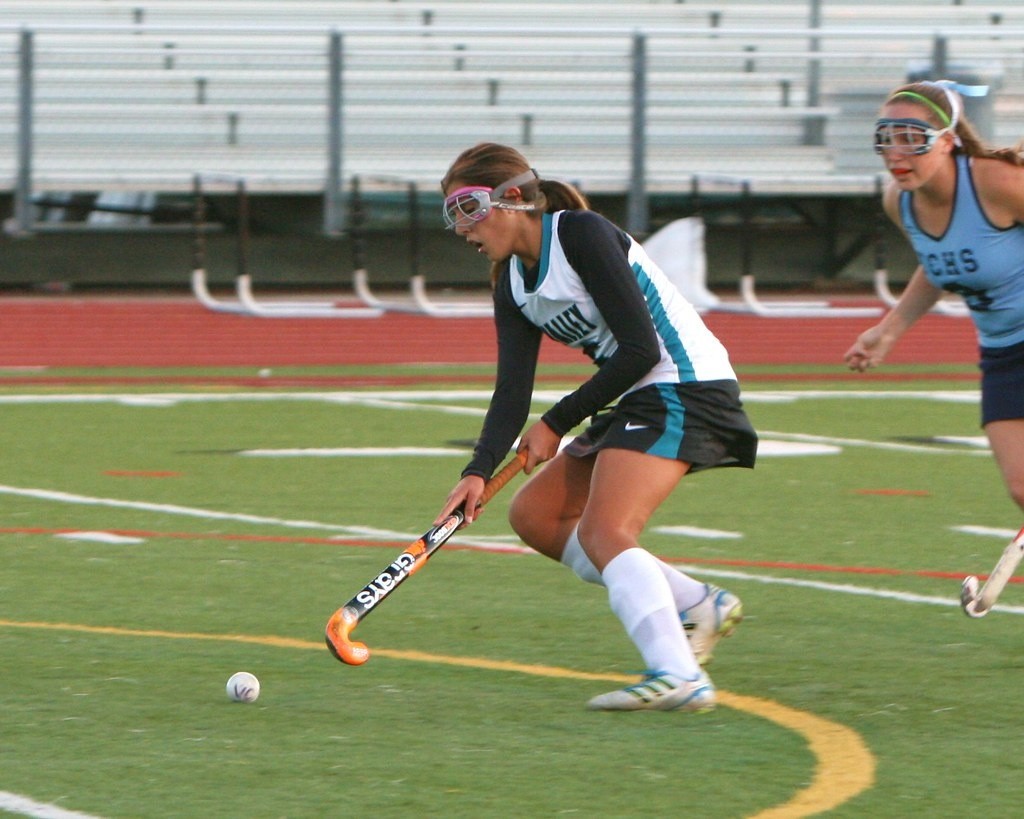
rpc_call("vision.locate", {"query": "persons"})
[429,142,762,717]
[841,81,1024,516]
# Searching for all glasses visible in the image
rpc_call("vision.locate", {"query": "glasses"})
[870,115,940,156]
[442,185,494,227]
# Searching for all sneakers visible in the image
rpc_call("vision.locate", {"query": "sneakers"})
[583,668,717,713]
[677,582,746,666]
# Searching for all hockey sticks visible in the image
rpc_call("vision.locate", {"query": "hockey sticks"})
[324,444,529,666]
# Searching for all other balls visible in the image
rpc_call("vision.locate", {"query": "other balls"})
[225,672,260,703]
